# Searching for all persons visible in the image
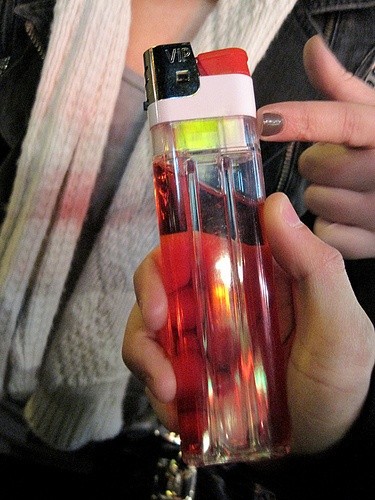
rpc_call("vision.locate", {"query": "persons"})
[0,0,375,500]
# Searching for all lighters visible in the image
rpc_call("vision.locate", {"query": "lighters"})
[142,41,293,467]
